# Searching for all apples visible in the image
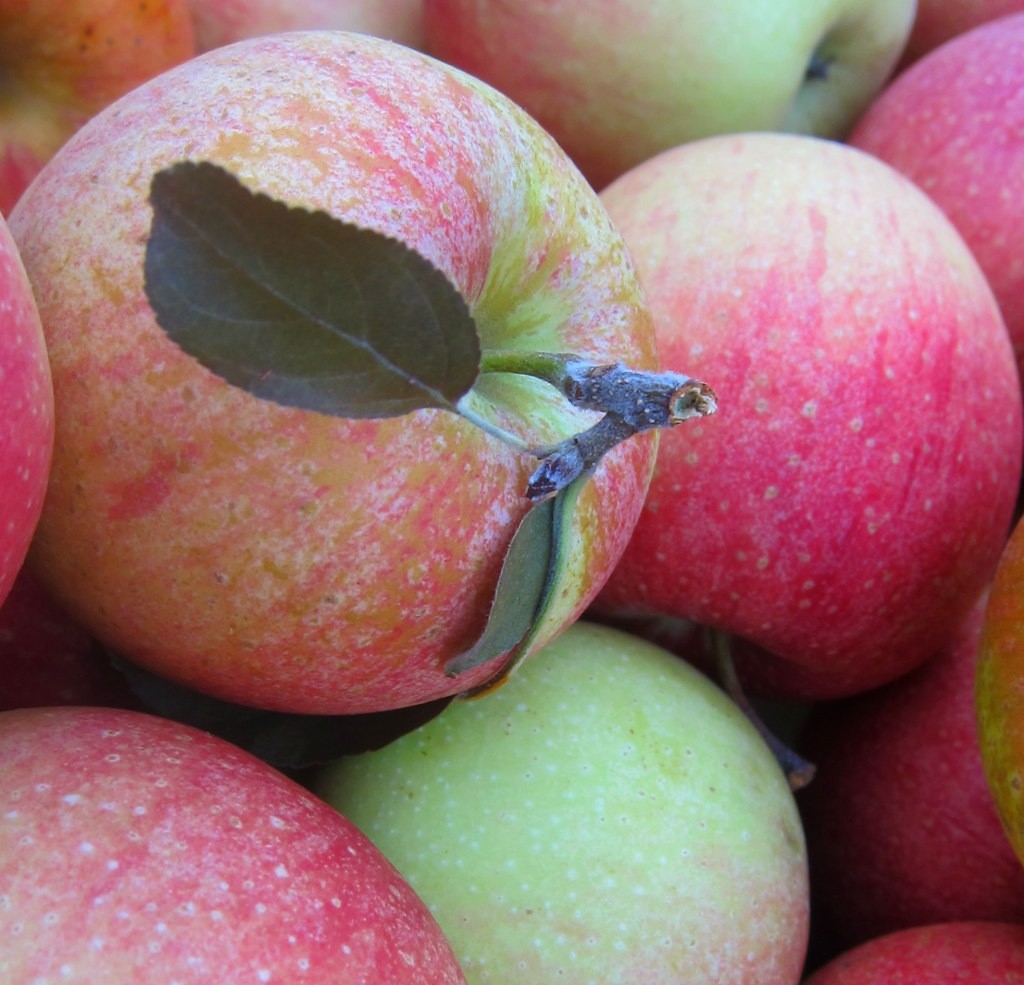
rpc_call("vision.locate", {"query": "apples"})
[0,1,1024,985]
